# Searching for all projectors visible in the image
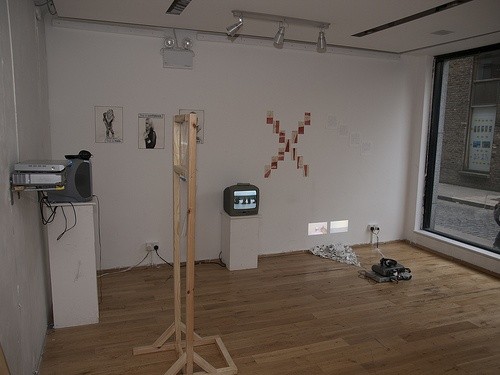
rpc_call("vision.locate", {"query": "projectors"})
[12,169,68,186]
[371,262,406,275]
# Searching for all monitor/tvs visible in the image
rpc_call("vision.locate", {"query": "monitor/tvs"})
[47,159,92,203]
[224,185,259,217]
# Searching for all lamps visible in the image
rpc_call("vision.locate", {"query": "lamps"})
[273,23,287,45]
[224,16,243,37]
[162,38,195,69]
[318,32,326,50]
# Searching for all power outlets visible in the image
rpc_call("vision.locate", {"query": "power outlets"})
[145,242,159,252]
[367,224,381,232]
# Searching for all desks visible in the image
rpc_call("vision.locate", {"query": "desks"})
[220,210,262,271]
[46,200,100,329]
